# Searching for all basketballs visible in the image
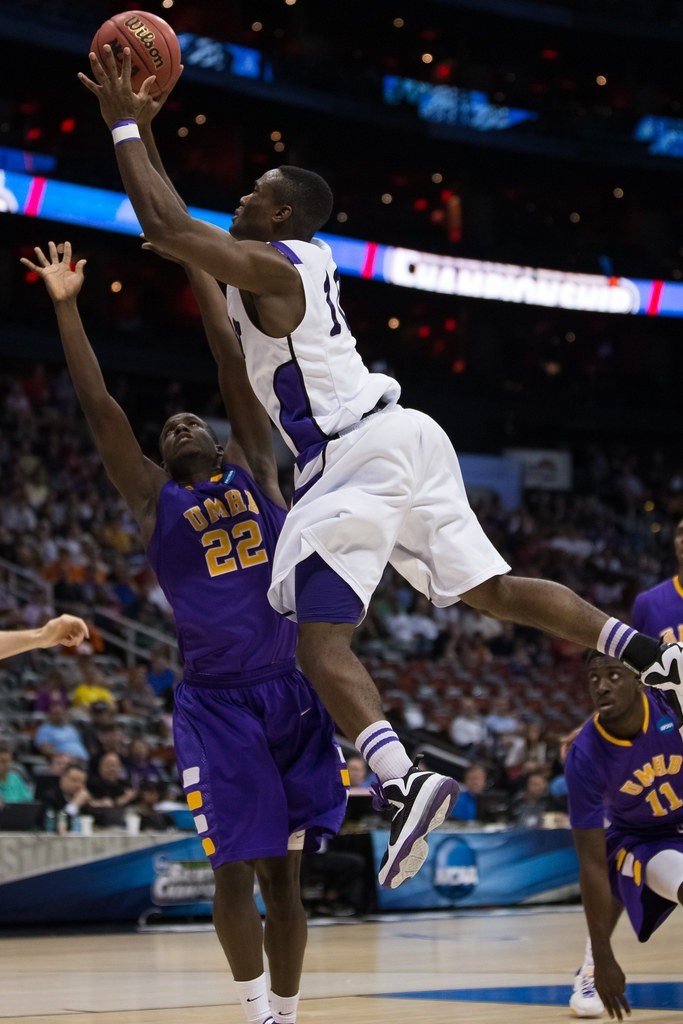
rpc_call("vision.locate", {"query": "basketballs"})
[89,10,181,98]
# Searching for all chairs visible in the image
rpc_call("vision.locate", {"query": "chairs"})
[1,653,159,776]
[356,654,593,742]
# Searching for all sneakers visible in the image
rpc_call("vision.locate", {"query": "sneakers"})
[569,966,604,1017]
[369,754,460,889]
[635,633,683,738]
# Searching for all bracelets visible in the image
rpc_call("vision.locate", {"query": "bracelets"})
[109,119,139,146]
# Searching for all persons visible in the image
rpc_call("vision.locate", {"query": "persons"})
[0,0,683,1024]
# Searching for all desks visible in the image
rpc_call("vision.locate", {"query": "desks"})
[0,832,267,933]
[326,825,582,916]
